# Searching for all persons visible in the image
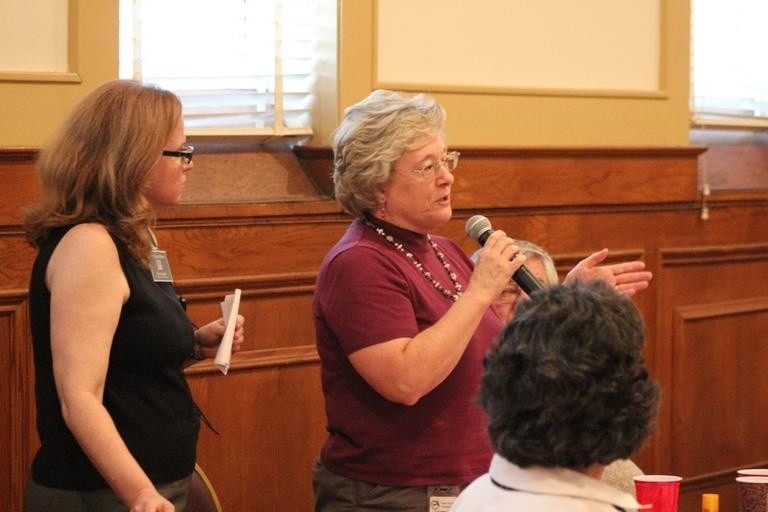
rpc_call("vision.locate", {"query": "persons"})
[438,277,659,510]
[19,80,246,511]
[468,239,560,329]
[310,89,655,511]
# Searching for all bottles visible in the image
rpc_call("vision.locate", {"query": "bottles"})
[700,492,721,511]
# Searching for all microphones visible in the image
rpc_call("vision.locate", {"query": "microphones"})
[465,215,545,299]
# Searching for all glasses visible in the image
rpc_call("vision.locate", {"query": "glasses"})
[386,148,462,182]
[157,144,196,165]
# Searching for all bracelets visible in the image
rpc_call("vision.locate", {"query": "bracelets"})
[189,339,207,362]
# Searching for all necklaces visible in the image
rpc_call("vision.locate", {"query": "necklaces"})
[364,217,462,303]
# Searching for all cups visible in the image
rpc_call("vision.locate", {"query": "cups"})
[631,473,684,512]
[735,466,768,512]
